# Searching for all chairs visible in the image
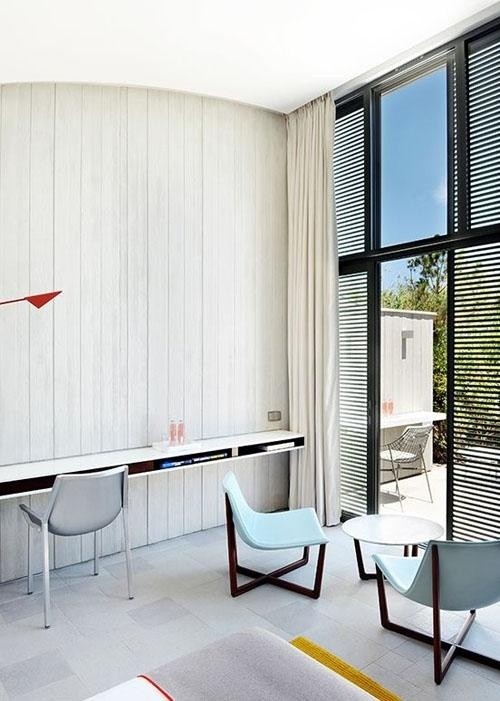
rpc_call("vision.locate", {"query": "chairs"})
[379,425,436,507]
[372,539,500,686]
[18,465,134,629]
[221,471,329,599]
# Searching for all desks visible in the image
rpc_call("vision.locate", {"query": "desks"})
[342,514,444,580]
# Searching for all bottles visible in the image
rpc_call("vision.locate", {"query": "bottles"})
[177,418,186,445]
[169,419,178,445]
